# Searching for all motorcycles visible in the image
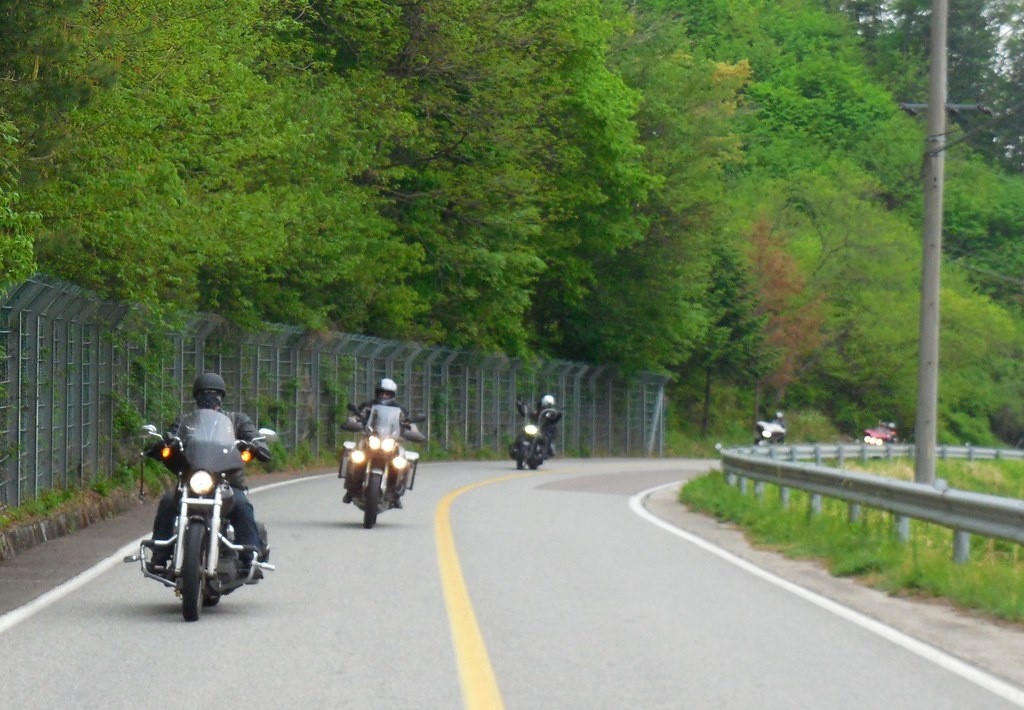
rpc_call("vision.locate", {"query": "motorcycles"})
[338,402,427,529]
[124,409,278,621]
[753,421,786,446]
[509,402,562,470]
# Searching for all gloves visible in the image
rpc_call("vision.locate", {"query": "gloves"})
[254,446,273,463]
[144,443,160,457]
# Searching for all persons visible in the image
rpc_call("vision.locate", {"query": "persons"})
[338,378,419,509]
[144,373,272,579]
[754,412,786,446]
[509,395,557,460]
[875,422,896,442]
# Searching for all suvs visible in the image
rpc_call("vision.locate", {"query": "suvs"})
[863,421,898,445]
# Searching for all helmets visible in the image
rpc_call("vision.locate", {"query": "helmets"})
[775,412,783,421]
[541,395,555,408]
[374,378,398,404]
[193,373,226,399]
[887,423,895,430]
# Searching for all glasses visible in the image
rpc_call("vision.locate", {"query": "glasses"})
[198,390,220,398]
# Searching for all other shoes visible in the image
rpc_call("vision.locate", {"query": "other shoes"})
[343,493,352,503]
[243,567,263,579]
[395,497,401,508]
[141,561,167,574]
